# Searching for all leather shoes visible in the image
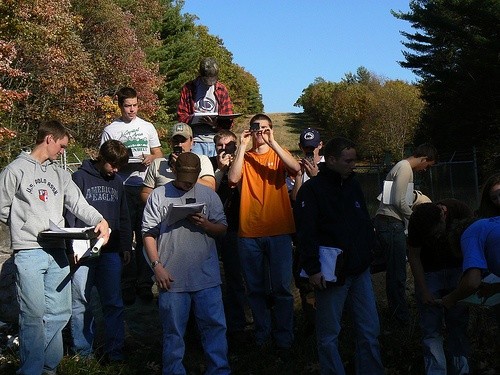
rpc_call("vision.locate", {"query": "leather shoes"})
[256,343,298,362]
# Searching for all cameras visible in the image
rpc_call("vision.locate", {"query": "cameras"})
[173,146,182,152]
[249,123,260,131]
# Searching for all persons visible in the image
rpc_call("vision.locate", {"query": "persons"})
[438,216,500,308]
[374,146,436,344]
[177,58,234,157]
[296,136,385,375]
[209,129,253,353]
[100,87,163,267]
[406,198,475,375]
[286,127,327,328]
[141,152,232,375]
[0,119,110,375]
[63,140,134,365]
[140,122,217,202]
[227,114,301,367]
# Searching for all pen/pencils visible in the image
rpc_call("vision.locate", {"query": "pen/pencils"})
[142,152,144,158]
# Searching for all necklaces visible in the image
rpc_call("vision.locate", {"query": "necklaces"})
[174,181,195,204]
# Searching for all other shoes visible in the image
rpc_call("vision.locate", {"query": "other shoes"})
[75,352,94,360]
[108,352,123,362]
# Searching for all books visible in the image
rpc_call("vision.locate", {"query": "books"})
[382,180,414,206]
[299,246,344,282]
[71,239,91,265]
[192,112,244,119]
[128,157,144,163]
[166,203,205,226]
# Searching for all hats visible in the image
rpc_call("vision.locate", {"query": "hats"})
[176,152,201,183]
[300,128,320,149]
[169,123,193,140]
[200,59,219,86]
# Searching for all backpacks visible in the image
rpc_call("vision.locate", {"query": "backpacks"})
[360,203,406,269]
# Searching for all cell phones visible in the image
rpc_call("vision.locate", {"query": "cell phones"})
[305,151,314,166]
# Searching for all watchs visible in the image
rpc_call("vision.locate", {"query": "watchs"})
[151,259,161,268]
